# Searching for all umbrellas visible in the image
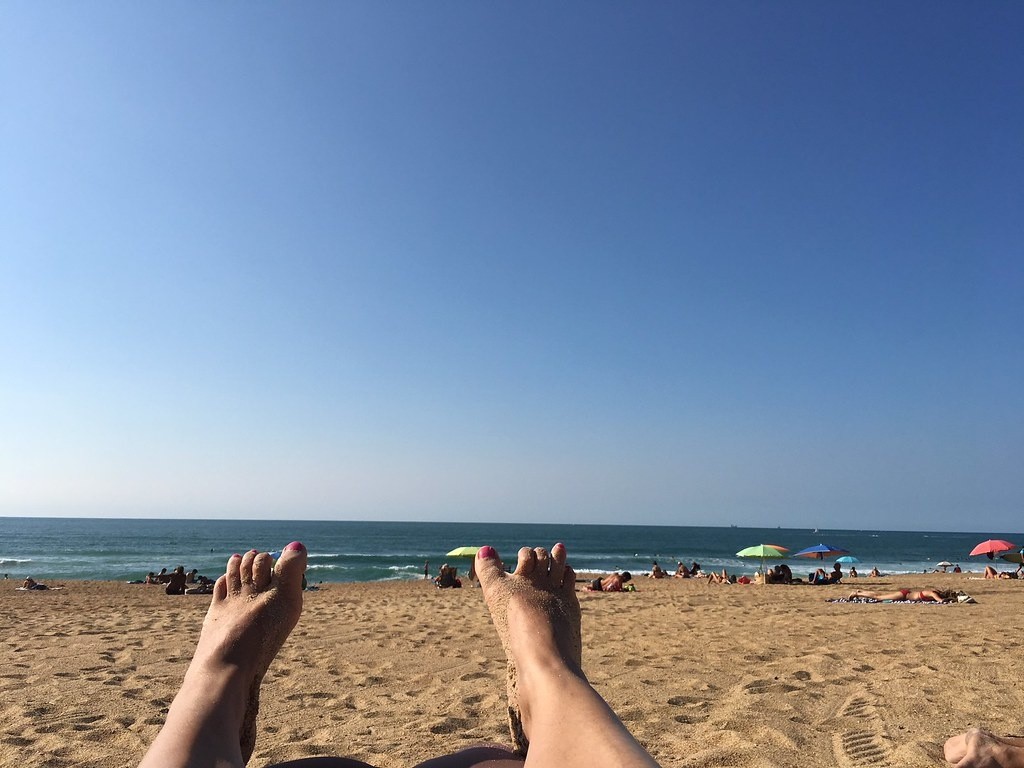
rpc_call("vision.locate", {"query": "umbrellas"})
[970,539,1016,571]
[836,555,859,564]
[937,561,954,567]
[445,546,484,579]
[736,544,789,572]
[793,543,850,571]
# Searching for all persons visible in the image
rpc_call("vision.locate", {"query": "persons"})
[923,546,1024,579]
[423,560,429,579]
[587,572,631,592]
[868,567,880,578]
[848,588,959,603]
[648,560,736,584]
[137,540,662,768]
[301,573,307,590]
[5,566,212,595]
[767,564,843,585]
[849,566,857,578]
